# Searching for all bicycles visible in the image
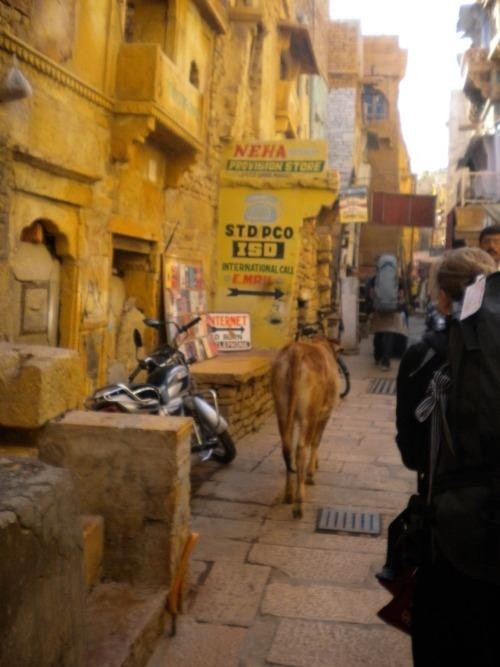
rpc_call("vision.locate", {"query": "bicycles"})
[296,321,351,398]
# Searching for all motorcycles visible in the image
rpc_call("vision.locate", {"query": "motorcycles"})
[82,317,237,464]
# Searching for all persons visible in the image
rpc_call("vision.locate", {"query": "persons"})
[392,270,500,667]
[478,227,500,269]
[367,269,430,371]
[434,246,497,327]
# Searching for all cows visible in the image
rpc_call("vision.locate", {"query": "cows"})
[270,331,350,520]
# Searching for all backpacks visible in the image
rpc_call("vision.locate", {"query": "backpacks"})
[371,266,399,312]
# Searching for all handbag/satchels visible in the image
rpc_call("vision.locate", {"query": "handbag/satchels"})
[375,373,498,593]
